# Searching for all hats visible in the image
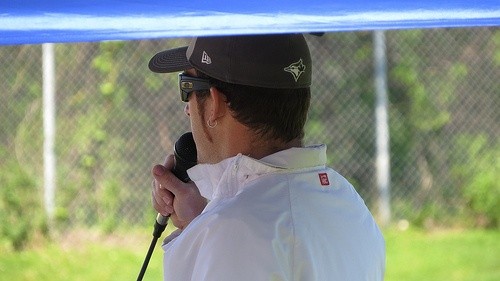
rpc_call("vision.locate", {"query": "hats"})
[148,34,313,90]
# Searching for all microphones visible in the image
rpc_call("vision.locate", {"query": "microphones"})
[153,132,198,238]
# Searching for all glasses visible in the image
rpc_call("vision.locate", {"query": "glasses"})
[178,73,212,103]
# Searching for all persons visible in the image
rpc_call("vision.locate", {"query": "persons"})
[148,33,385,281]
